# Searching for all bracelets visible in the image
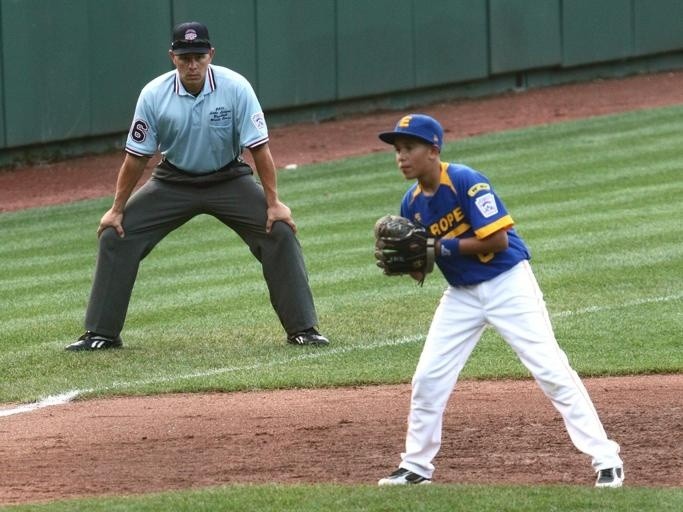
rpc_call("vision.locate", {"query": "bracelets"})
[437,236,461,258]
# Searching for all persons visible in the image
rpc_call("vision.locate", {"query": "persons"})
[374,114,629,494]
[62,21,331,353]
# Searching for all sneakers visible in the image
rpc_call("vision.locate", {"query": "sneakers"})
[377,468,431,486]
[64,330,122,351]
[287,326,329,346]
[594,467,625,488]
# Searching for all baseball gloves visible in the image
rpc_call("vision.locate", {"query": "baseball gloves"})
[374,213,435,288]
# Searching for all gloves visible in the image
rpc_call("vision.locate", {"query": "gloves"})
[374,213,435,287]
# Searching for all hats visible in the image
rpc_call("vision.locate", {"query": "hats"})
[378,114,443,152]
[171,22,211,55]
